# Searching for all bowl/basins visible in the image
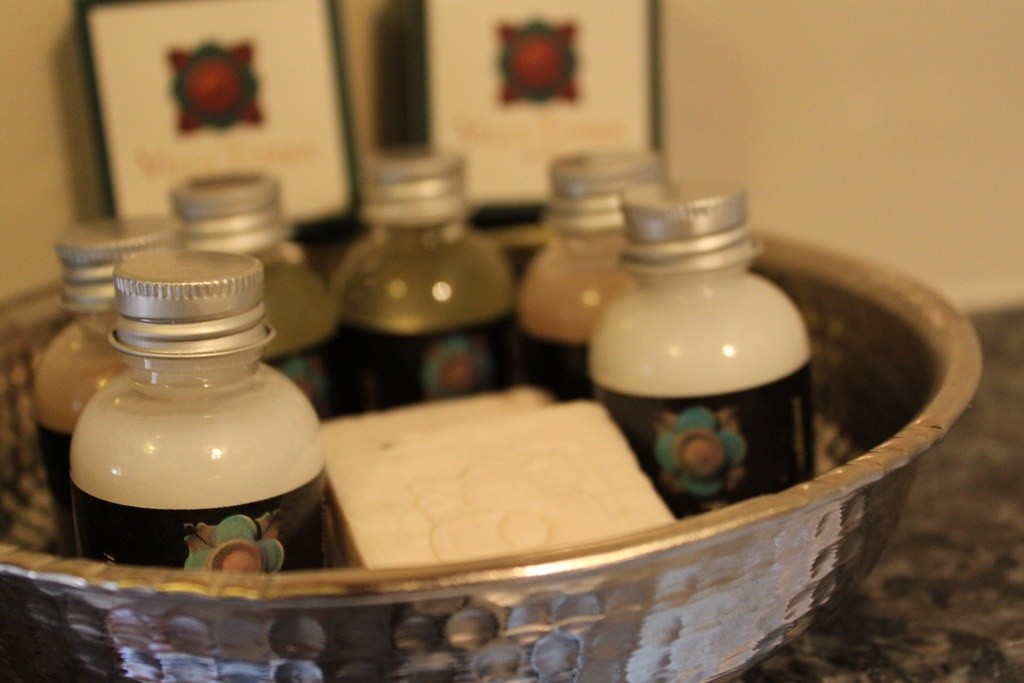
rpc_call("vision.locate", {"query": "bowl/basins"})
[0,201,982,683]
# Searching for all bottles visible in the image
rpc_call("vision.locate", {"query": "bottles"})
[587,184,819,514]
[520,151,668,399]
[331,150,516,414]
[36,224,198,555]
[173,176,334,421]
[68,254,327,568]
